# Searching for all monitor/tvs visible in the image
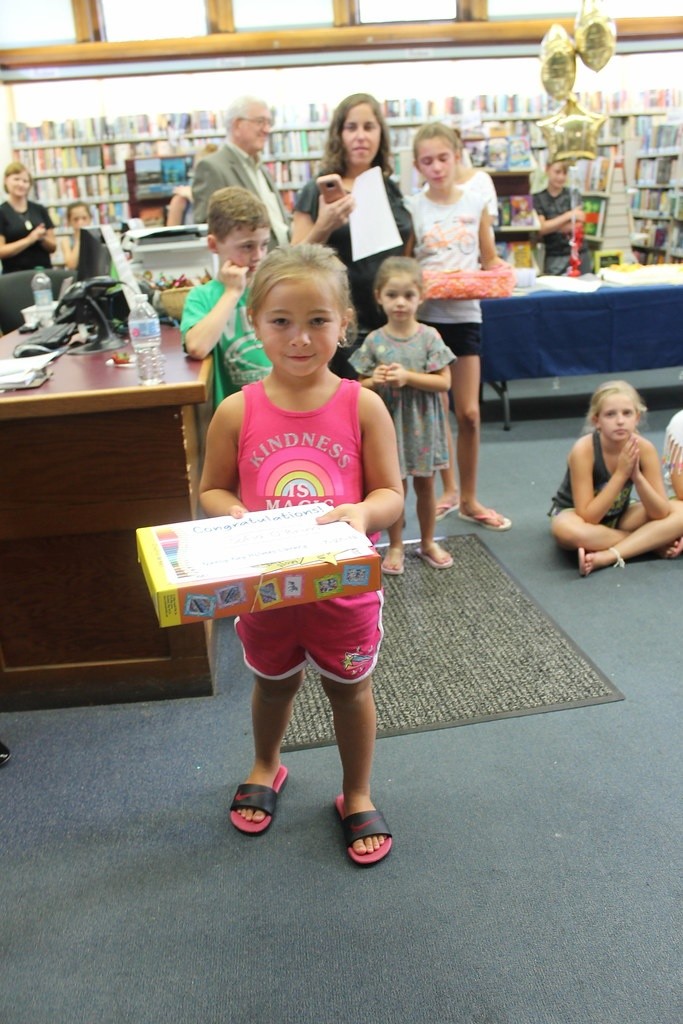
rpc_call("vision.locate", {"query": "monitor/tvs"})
[67,223,131,355]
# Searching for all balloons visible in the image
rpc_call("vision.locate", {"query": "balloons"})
[577,0,618,73]
[537,95,608,163]
[541,25,577,101]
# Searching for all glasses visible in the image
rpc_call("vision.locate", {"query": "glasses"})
[242,114,273,129]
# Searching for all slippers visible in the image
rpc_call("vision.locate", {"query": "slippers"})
[230,766,289,835]
[381,548,406,574]
[435,497,460,522]
[458,506,512,531]
[578,546,588,576]
[415,545,453,569]
[335,793,392,867]
[658,538,683,559]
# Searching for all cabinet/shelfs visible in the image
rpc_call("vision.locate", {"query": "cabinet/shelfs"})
[13,105,683,285]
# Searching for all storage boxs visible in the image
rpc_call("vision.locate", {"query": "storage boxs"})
[135,502,381,628]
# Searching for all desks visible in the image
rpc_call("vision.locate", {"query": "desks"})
[473,274,683,430]
[0,324,216,715]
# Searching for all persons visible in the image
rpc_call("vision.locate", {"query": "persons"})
[192,99,293,254]
[166,144,217,226]
[533,160,584,276]
[547,381,683,576]
[347,255,453,575]
[290,93,415,381]
[402,122,513,532]
[0,164,58,277]
[178,186,272,395]
[60,205,92,272]
[197,245,405,866]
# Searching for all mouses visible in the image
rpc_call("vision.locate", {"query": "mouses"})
[14,344,51,358]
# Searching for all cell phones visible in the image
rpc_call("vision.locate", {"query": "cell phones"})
[315,173,346,203]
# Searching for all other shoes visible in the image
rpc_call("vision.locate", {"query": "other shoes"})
[0,740,12,766]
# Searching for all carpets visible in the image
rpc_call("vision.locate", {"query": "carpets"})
[279,533,625,754]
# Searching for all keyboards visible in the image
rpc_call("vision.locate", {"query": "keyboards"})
[22,322,76,349]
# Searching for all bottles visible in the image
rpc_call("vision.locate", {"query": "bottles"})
[127,294,165,384]
[31,266,52,306]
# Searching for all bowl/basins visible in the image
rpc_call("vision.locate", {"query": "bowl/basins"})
[21,301,59,324]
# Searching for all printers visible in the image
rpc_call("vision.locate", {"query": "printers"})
[126,223,220,288]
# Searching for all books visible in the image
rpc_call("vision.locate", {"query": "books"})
[12,85,683,274]
